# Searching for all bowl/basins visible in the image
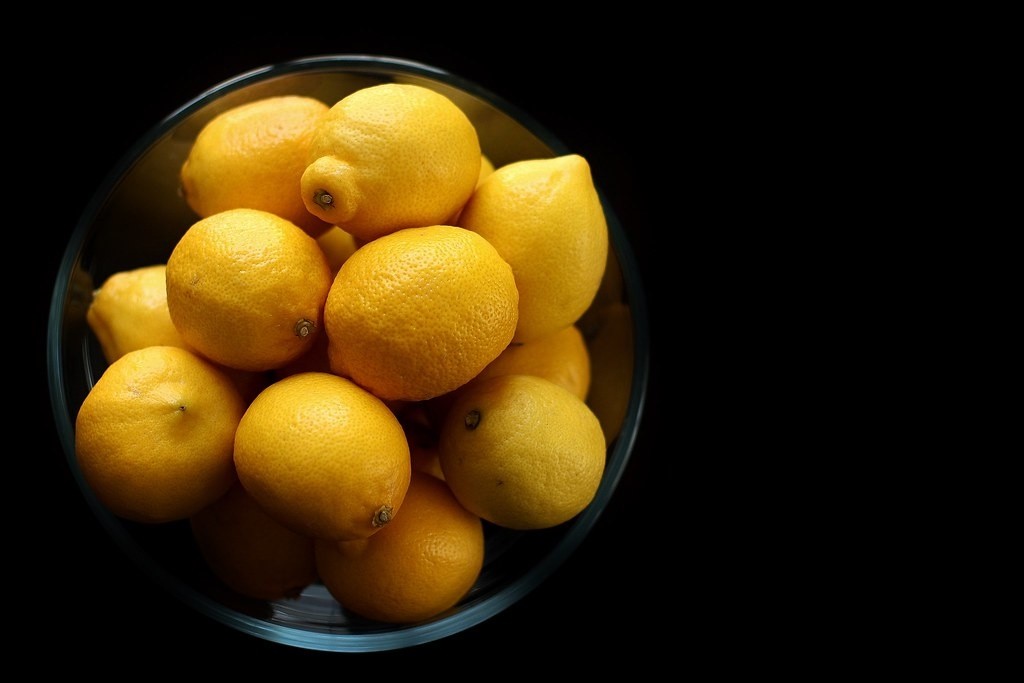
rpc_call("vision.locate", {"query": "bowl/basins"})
[52,57,653,653]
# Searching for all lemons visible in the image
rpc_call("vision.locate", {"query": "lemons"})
[73,84,608,623]
